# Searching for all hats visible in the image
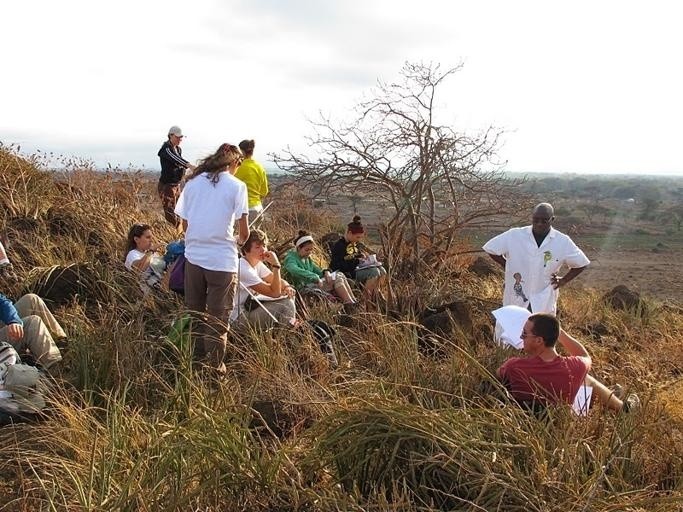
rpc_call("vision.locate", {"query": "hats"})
[167,125,183,138]
[6,363,45,413]
[355,252,383,272]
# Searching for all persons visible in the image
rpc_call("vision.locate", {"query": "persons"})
[480,200,591,321]
[492,312,639,426]
[0,244,17,285]
[232,139,270,231]
[155,126,197,232]
[120,215,388,340]
[171,142,249,387]
[0,290,72,379]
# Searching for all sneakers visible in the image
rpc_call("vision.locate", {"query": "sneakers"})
[606,382,624,401]
[627,392,642,414]
[343,300,363,314]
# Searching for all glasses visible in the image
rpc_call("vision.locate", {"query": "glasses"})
[520,329,536,338]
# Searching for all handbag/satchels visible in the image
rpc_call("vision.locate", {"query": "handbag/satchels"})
[169,254,187,294]
